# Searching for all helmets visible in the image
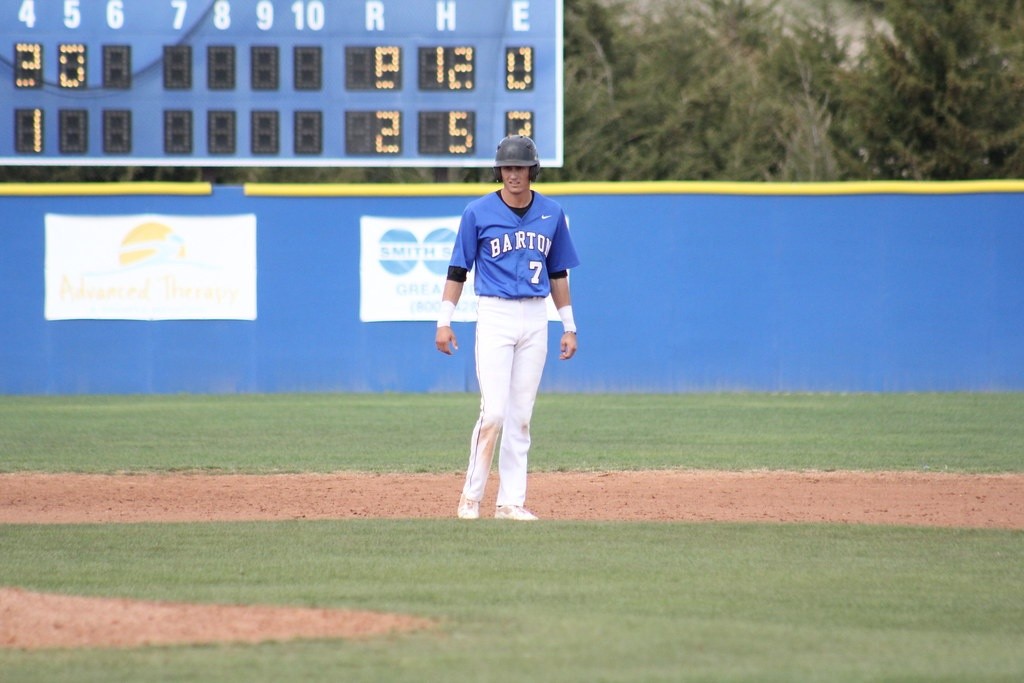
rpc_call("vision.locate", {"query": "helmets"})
[493,135,540,183]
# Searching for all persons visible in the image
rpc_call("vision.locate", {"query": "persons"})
[435,135,582,520]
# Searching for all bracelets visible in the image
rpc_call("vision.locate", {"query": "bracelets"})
[558,305,577,334]
[437,300,455,328]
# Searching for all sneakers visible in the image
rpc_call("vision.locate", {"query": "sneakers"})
[496,505,539,521]
[458,493,480,520]
